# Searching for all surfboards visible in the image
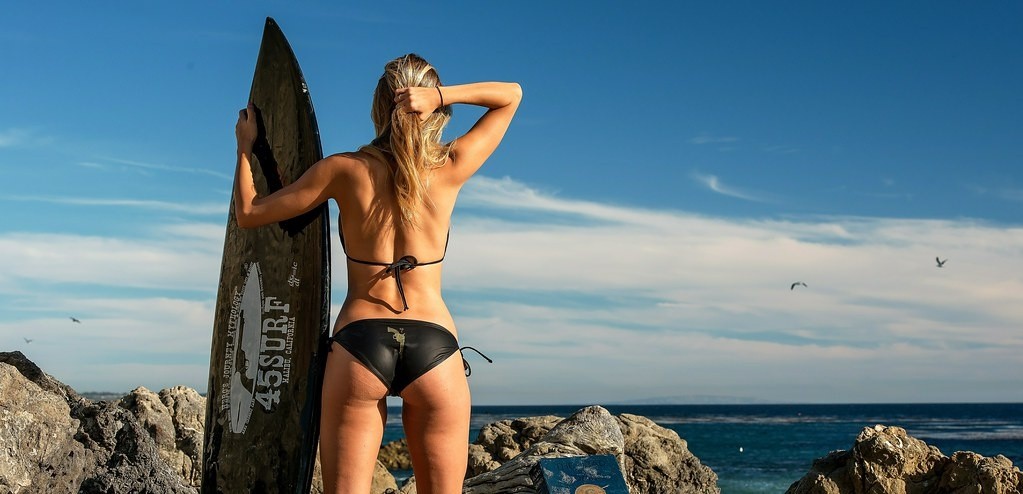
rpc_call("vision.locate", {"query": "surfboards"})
[197,12,332,494]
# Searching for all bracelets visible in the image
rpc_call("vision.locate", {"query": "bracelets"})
[434,85,443,108]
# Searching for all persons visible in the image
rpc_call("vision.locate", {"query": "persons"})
[236,53,523,494]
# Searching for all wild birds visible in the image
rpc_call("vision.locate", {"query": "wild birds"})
[937,257,946,267]
[791,282,807,290]
[71,317,81,323]
[24,338,32,342]
